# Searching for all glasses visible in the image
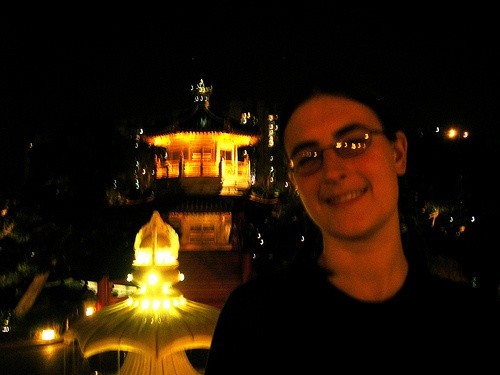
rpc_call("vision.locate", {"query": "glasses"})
[286,128,389,177]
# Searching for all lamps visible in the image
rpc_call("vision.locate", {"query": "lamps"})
[126,210,187,310]
